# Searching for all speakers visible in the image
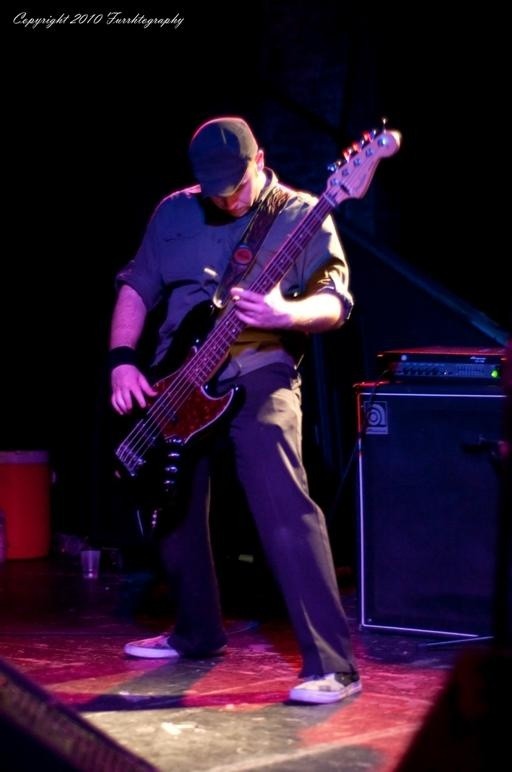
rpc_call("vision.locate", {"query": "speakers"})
[358,385,512,637]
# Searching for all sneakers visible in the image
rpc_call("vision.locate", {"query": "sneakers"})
[124,631,227,658]
[290,672,362,704]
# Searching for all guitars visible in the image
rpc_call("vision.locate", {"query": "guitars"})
[85,125,402,534]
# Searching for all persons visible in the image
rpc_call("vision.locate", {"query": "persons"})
[108,116,364,703]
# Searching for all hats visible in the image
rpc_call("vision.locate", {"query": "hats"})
[188,116,258,198]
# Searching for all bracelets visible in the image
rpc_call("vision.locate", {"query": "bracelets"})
[110,346,141,368]
